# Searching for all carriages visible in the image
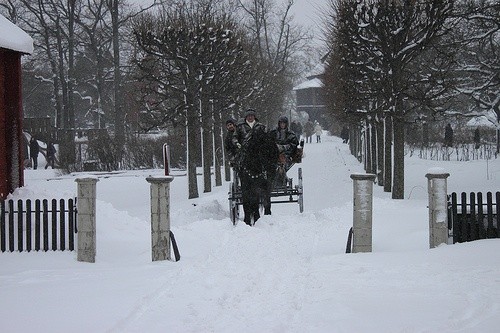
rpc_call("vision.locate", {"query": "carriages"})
[226,126,304,226]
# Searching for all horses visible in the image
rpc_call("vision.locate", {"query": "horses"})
[236,124,279,227]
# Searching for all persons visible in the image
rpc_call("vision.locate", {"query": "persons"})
[474,129,481,148]
[315,122,322,143]
[232,109,266,149]
[304,119,314,143]
[225,120,240,170]
[296,122,302,140]
[341,126,349,144]
[276,115,299,168]
[442,123,453,147]
[290,120,297,134]
[30,136,38,170]
[45,141,56,169]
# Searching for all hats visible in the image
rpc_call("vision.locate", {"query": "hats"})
[245,108,259,122]
[226,120,234,126]
[278,116,289,128]
